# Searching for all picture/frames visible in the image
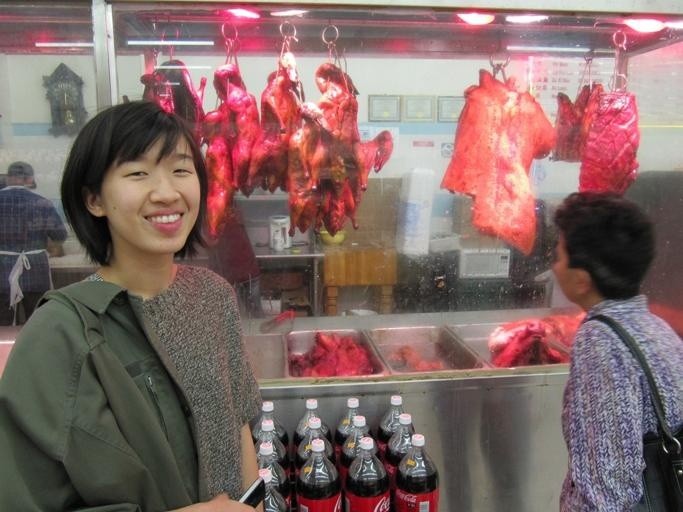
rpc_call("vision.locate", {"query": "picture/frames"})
[368,94,401,122]
[438,95,466,123]
[402,94,438,122]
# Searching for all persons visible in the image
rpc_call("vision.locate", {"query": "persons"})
[0,100,266,512]
[554,190,683,512]
[0,161,68,327]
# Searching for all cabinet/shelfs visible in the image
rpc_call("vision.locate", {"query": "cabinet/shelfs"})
[0,305,586,512]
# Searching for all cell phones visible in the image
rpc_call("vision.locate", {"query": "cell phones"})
[237,477,266,507]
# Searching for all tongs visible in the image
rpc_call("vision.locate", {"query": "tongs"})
[260,308,298,338]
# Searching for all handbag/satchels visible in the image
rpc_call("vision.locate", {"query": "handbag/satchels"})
[580,314,683,511]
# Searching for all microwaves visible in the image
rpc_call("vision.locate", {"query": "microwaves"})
[459,245,510,279]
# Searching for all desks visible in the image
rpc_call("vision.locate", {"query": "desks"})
[322,234,398,315]
[48,242,324,318]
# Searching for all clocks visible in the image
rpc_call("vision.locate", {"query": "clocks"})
[42,62,88,137]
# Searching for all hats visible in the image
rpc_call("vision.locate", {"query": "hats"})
[9,162,37,189]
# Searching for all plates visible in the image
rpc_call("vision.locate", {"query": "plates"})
[285,329,388,381]
[246,330,287,385]
[445,321,576,370]
[365,325,490,376]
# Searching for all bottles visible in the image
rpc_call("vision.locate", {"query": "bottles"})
[252,401,290,458]
[376,395,405,463]
[295,417,336,482]
[344,437,390,512]
[394,434,439,512]
[385,413,416,504]
[335,397,373,468]
[259,469,288,512]
[296,439,342,512]
[294,398,333,453]
[340,416,383,493]
[255,419,290,480]
[257,442,291,507]
